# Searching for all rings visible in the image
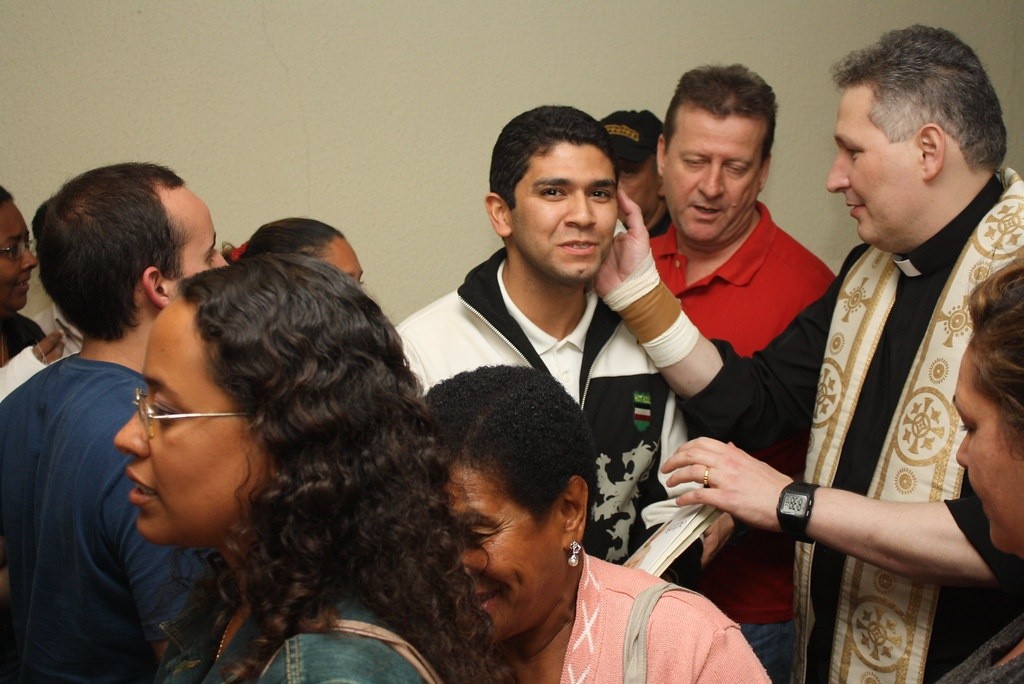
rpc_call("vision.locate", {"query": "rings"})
[703,468,709,485]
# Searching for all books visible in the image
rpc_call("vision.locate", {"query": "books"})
[622,504,724,577]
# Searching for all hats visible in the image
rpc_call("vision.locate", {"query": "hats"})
[600,109,664,163]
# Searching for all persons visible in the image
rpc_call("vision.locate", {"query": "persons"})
[113,254,506,684]
[935,263,1024,684]
[599,109,672,239]
[0,188,66,403]
[0,162,229,684]
[623,520,672,569]
[395,106,734,589]
[425,365,772,684]
[647,64,837,684]
[592,25,1024,684]
[221,217,363,284]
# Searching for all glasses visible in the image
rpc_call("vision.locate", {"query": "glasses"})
[132,384,259,438]
[0,239,38,260]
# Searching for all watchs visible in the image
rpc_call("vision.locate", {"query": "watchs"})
[776,481,820,544]
